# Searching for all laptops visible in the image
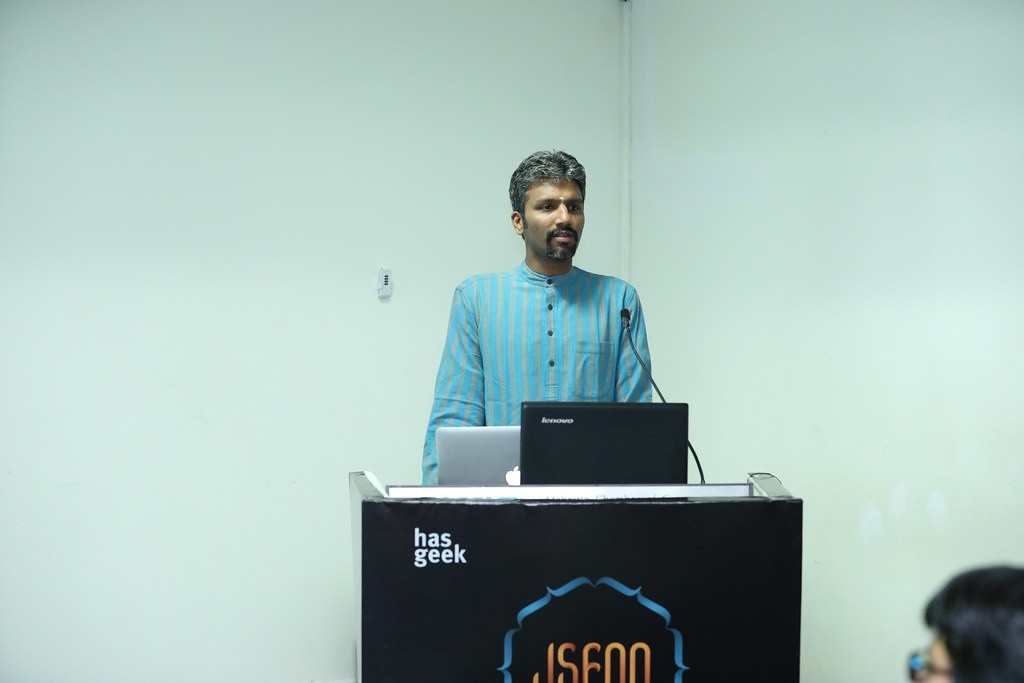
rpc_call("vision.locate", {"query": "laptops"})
[520,401,689,484]
[435,426,521,487]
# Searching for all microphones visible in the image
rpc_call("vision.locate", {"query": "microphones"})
[620,309,706,484]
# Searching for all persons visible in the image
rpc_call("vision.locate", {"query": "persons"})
[422,151,653,486]
[907,565,1024,683]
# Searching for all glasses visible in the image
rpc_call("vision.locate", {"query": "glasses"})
[904,648,949,683]
